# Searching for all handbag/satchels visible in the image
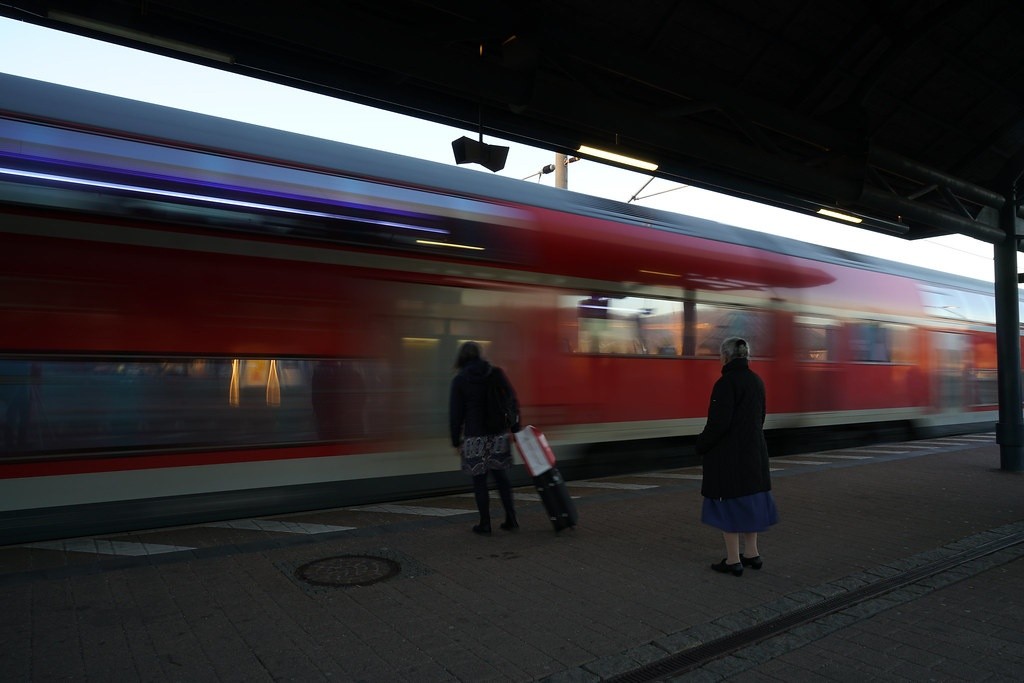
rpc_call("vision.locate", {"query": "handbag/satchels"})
[513,425,556,477]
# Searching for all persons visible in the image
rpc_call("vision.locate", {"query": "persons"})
[687,336,781,579]
[447,340,524,535]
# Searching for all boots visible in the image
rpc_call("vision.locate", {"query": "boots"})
[473,514,493,538]
[499,511,519,530]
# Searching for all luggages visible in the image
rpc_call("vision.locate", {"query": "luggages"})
[534,469,579,535]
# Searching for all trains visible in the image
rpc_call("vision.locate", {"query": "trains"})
[0,71,1023,548]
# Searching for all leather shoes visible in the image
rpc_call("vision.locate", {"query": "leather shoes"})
[740,553,761,569]
[712,558,743,576]
